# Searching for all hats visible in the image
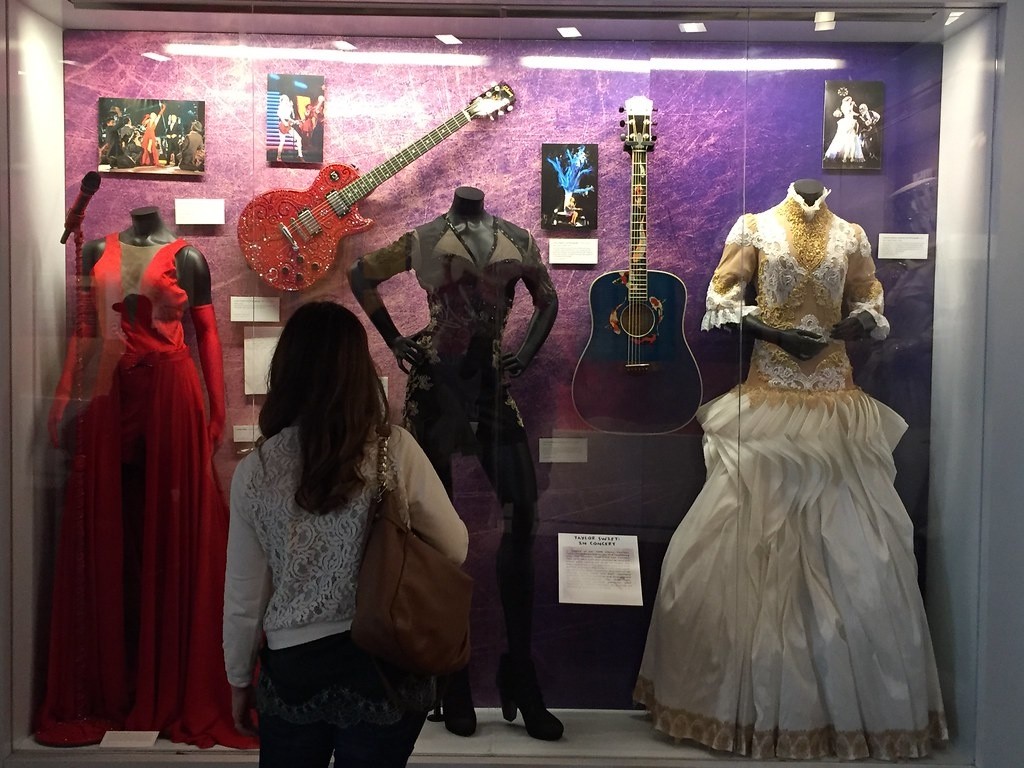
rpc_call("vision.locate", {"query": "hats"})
[191,120,202,132]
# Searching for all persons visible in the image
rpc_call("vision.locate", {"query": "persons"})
[565,197,582,226]
[223,300,471,768]
[349,187,565,741]
[31,204,260,746]
[102,103,205,170]
[633,178,949,762]
[278,93,307,165]
[824,96,881,163]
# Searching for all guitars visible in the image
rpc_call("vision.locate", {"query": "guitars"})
[235,80,516,295]
[570,92,703,440]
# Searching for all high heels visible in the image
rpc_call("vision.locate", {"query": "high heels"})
[495,653,564,743]
[434,672,475,736]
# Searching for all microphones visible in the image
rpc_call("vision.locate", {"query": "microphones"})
[59,171,101,244]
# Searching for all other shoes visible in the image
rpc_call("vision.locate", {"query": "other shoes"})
[174,164,178,166]
[166,163,170,165]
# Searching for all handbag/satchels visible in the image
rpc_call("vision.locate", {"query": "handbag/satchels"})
[351,427,473,674]
